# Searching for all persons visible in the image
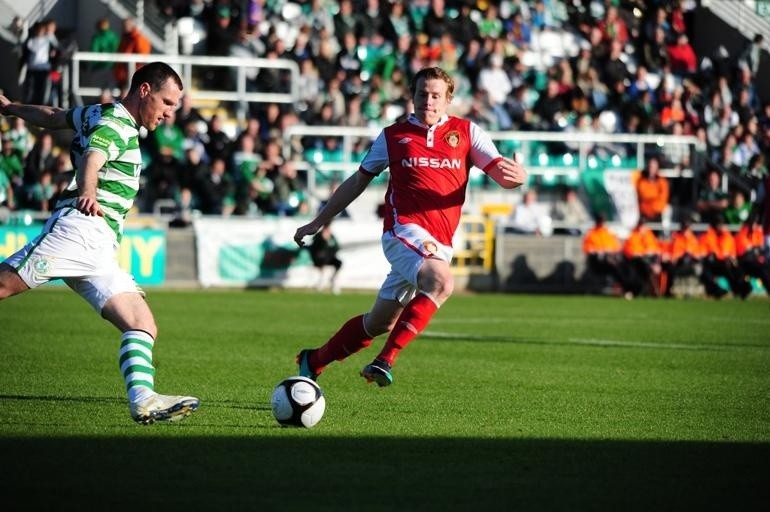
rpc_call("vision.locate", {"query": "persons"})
[0,0,770,300]
[293,67,528,387]
[0,61,200,425]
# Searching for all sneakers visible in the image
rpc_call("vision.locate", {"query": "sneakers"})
[131,394,200,425]
[296,349,322,382]
[360,359,392,387]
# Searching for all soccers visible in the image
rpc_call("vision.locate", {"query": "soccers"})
[270,376,325,428]
